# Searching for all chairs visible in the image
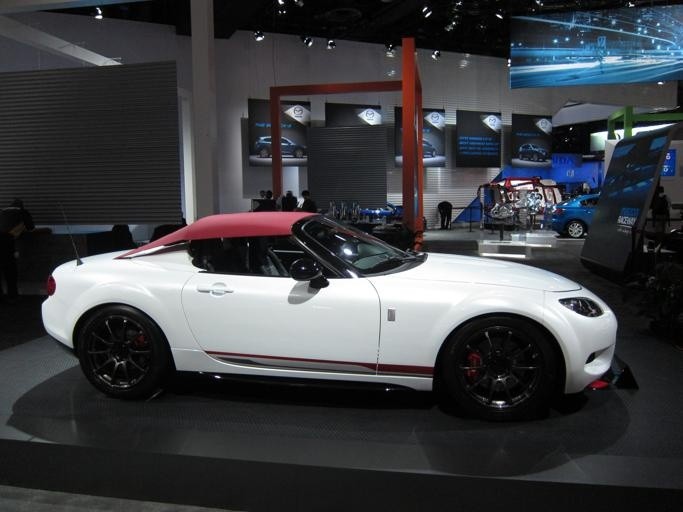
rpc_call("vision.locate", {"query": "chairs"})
[253,247,288,276]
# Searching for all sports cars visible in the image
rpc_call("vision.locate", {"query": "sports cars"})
[38,207,646,425]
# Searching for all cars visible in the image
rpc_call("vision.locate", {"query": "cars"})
[542,192,600,239]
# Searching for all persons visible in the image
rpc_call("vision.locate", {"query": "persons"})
[258,190,265,199]
[298,190,318,213]
[659,186,672,213]
[649,192,671,233]
[0,198,37,308]
[256,190,277,213]
[279,191,298,212]
[436,201,453,231]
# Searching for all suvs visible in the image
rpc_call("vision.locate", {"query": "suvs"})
[252,134,307,159]
[422,138,437,157]
[518,143,548,162]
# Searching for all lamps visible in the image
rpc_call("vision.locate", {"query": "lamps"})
[327,39,336,51]
[278,0,287,14]
[628,2,635,8]
[432,50,442,63]
[253,28,265,42]
[422,6,432,18]
[303,36,314,49]
[496,8,504,19]
[444,1,462,31]
[385,42,395,58]
[94,7,103,21]
